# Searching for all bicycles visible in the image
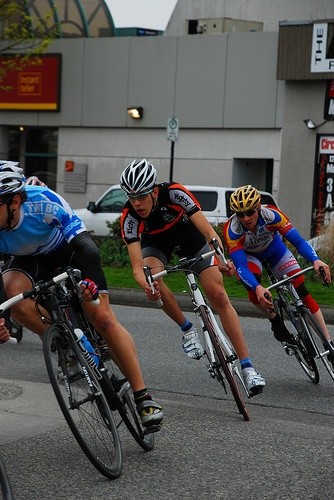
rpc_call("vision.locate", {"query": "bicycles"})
[0,266,156,479]
[142,237,259,422]
[247,256,334,385]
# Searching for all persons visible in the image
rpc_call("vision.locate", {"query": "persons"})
[0,160,164,426]
[120,158,266,390]
[222,184,334,370]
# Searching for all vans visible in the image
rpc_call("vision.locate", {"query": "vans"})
[73,184,284,240]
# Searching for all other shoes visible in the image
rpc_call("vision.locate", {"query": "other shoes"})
[326,350,334,369]
[270,321,291,341]
[5,320,24,344]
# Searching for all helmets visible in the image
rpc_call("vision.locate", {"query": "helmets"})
[26,176,47,187]
[119,158,158,196]
[0,159,26,195]
[230,184,260,212]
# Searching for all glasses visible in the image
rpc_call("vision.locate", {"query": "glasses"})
[125,189,154,202]
[236,206,258,219]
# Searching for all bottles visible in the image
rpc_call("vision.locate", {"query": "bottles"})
[290,300,300,320]
[74,329,99,366]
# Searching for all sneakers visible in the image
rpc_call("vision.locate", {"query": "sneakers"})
[55,344,82,381]
[241,369,265,392]
[135,401,164,426]
[182,326,204,358]
